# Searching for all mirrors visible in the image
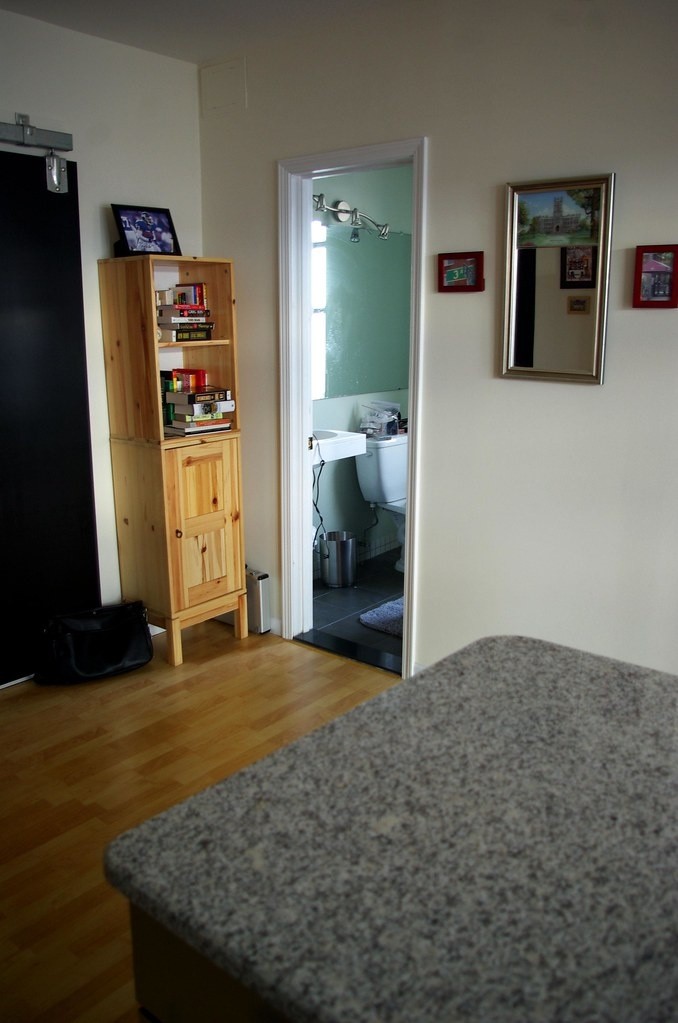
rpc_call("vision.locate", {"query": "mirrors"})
[499,171,619,388]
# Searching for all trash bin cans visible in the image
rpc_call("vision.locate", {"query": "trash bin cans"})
[318,530,357,588]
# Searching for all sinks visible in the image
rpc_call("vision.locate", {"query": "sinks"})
[313,430,367,464]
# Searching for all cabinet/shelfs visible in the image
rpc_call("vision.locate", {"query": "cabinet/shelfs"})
[97,251,248,669]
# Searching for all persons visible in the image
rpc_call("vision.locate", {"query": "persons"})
[135,212,172,252]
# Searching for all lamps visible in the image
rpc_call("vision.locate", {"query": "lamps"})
[311,193,390,243]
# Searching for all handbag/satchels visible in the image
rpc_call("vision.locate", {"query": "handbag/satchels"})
[34,601,154,686]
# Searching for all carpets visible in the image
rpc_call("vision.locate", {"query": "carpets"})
[360,596,404,636]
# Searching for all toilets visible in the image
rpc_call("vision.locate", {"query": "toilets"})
[356,434,407,573]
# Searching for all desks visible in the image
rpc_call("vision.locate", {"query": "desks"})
[101,633,677,1023]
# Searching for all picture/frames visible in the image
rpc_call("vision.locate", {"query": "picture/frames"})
[632,244,678,308]
[437,250,486,293]
[566,295,592,316]
[109,202,183,256]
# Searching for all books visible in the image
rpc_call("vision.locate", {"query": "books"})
[161,369,235,437]
[155,282,216,342]
